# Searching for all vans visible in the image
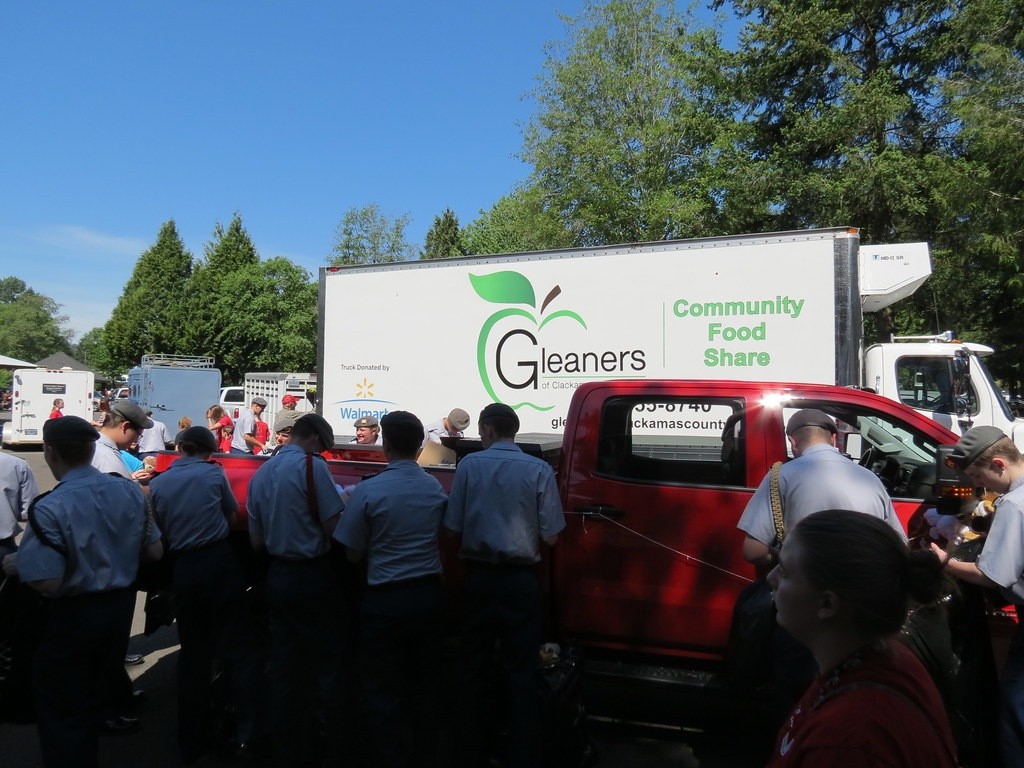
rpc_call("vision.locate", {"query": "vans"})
[109,388,128,404]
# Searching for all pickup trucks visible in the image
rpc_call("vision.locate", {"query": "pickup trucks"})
[140,378,1017,707]
[219,386,246,423]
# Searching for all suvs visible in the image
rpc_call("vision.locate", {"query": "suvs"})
[93,389,103,412]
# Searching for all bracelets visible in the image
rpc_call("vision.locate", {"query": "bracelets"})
[942,555,953,568]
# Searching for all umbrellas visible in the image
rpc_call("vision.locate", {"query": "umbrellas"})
[34,351,108,382]
[0,355,38,367]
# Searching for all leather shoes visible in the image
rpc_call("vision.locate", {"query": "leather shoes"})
[583,741,599,768]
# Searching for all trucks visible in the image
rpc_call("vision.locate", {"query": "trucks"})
[243,372,318,445]
[313,221,1024,470]
[127,352,223,450]
[2,367,96,446]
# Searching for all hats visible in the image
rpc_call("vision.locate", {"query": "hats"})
[43,416,100,442]
[448,408,470,430]
[112,400,154,428]
[175,426,218,452]
[303,414,334,448]
[380,411,424,441]
[354,416,378,427]
[478,403,519,434]
[275,419,296,431]
[786,409,838,436]
[282,395,300,404]
[252,397,267,405]
[952,426,1006,472]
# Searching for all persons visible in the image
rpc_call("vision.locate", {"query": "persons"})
[3,416,164,768]
[48,389,318,664]
[898,426,1024,768]
[0,447,38,729]
[420,408,470,448]
[0,390,12,410]
[443,403,566,768]
[736,409,909,712]
[246,414,346,768]
[332,411,450,768]
[349,416,383,444]
[766,509,958,768]
[148,427,239,767]
